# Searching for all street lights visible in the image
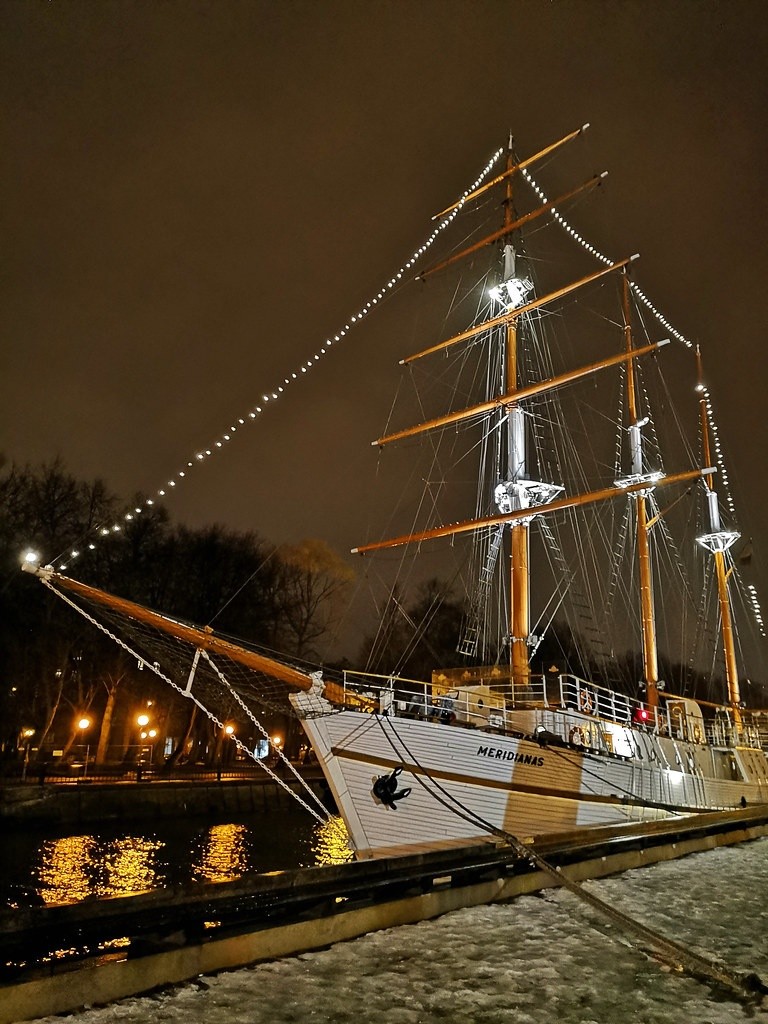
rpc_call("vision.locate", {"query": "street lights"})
[78,718,90,754]
[136,715,149,763]
[222,725,234,765]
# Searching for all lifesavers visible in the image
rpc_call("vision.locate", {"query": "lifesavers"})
[694,724,700,740]
[581,692,592,712]
[570,728,585,745]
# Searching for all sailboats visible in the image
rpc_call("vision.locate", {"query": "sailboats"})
[22,115,768,870]
